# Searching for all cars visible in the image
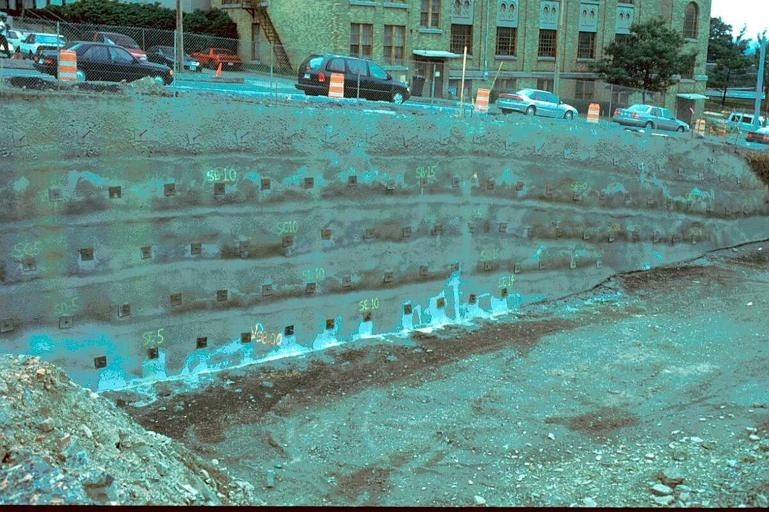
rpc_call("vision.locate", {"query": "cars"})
[746,126,769,150]
[0,30,243,85]
[496,88,578,120]
[613,104,689,133]
[295,53,412,104]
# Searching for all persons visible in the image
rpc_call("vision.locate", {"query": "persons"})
[0,13,14,60]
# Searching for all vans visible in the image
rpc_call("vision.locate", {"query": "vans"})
[725,113,769,132]
